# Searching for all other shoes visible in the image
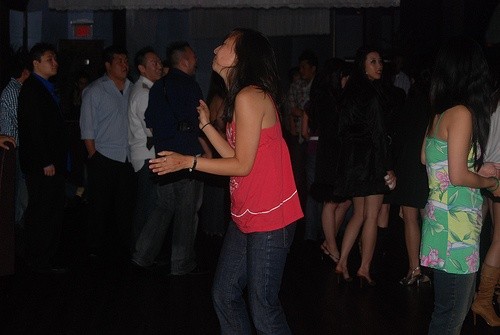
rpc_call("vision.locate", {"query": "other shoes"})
[86,247,100,263]
[172,264,212,276]
[129,255,163,272]
[199,232,224,240]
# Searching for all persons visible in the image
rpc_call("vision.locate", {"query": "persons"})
[0,0,500,335]
[149,28,304,335]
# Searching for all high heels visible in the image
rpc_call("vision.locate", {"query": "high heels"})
[318,244,340,265]
[335,264,355,287]
[356,272,380,289]
[400,267,421,287]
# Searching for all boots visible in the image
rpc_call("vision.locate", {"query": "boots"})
[377,225,393,271]
[471,261,500,328]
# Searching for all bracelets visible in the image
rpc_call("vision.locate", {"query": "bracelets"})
[201,122,211,130]
[486,176,499,191]
[189,153,196,172]
